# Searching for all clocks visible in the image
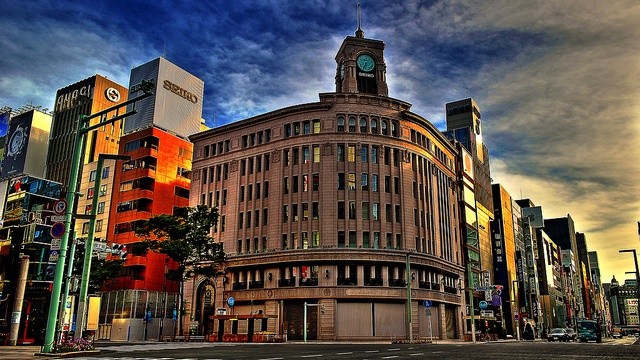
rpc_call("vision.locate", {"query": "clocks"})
[356,53,375,72]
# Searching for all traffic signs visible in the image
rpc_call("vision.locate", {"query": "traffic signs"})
[423,299,432,308]
[478,300,488,310]
[49,238,62,251]
[52,198,68,216]
[49,221,66,239]
[226,296,235,307]
[49,250,60,262]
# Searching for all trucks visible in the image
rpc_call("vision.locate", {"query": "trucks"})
[577,319,602,343]
[613,328,622,339]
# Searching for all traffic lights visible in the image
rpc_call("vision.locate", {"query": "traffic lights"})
[473,284,504,296]
[93,240,128,260]
[129,76,155,94]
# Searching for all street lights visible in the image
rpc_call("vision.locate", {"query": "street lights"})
[571,315,589,338]
[303,301,326,343]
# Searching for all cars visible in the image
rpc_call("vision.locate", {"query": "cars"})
[565,328,577,342]
[548,328,569,342]
